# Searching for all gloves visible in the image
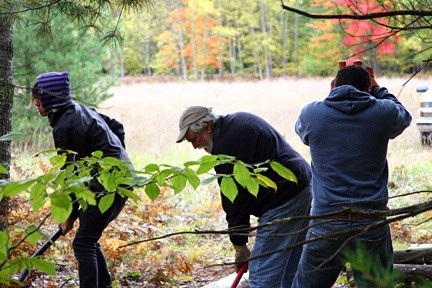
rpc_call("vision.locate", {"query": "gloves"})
[233,243,251,274]
[361,62,379,86]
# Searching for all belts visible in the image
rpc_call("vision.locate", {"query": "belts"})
[326,213,371,219]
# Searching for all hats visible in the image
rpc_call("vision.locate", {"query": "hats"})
[176,106,212,143]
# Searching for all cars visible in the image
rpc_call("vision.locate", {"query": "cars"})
[416,85,432,144]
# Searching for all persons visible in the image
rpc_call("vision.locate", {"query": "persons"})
[31,71,137,288]
[176,106,312,288]
[290,61,412,288]
